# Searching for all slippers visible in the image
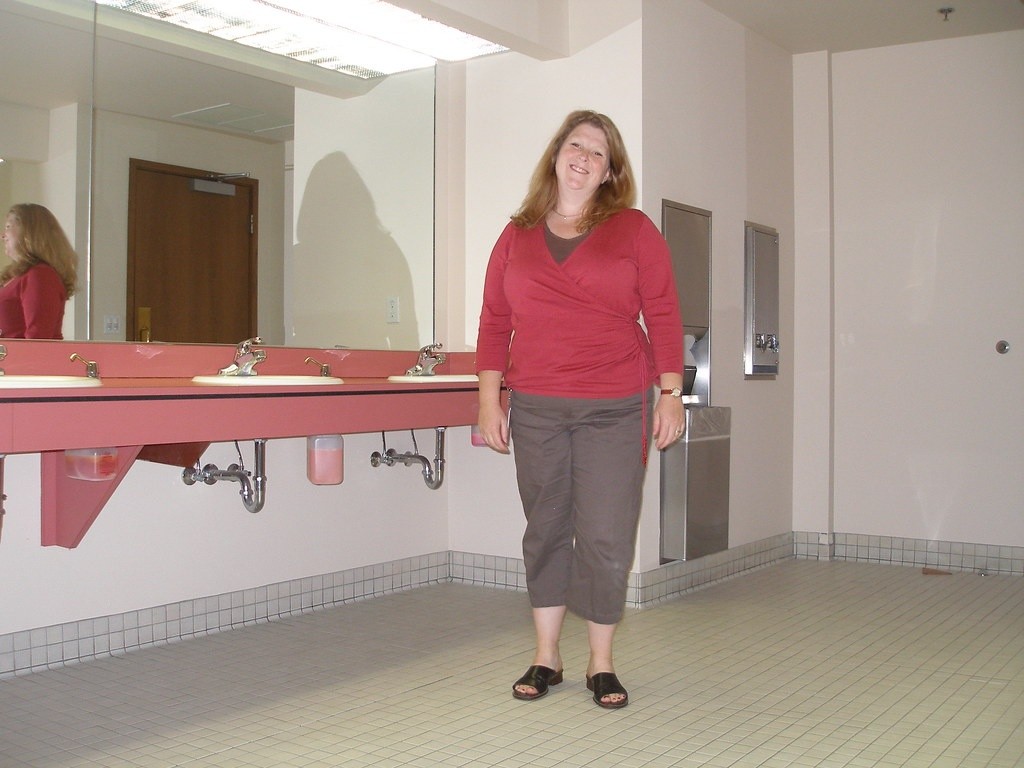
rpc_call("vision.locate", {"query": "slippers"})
[587,672,628,709]
[512,665,564,700]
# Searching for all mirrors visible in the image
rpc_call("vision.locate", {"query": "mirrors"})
[0,0,436,351]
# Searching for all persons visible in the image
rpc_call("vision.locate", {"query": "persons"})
[0,202,79,341]
[478,108,686,708]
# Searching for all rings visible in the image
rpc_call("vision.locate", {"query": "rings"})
[675,429,681,435]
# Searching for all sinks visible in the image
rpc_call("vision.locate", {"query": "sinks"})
[0,375,102,388]
[386,375,479,382]
[191,375,344,387]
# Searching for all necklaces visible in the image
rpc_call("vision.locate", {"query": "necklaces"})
[551,206,584,220]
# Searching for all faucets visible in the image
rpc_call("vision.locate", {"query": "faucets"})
[304,356,328,377]
[70,352,97,377]
[0,345,7,376]
[218,336,266,375]
[406,343,445,376]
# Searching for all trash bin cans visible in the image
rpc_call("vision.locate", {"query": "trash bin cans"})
[658,403,732,562]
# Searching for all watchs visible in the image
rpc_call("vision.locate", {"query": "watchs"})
[660,387,684,397]
